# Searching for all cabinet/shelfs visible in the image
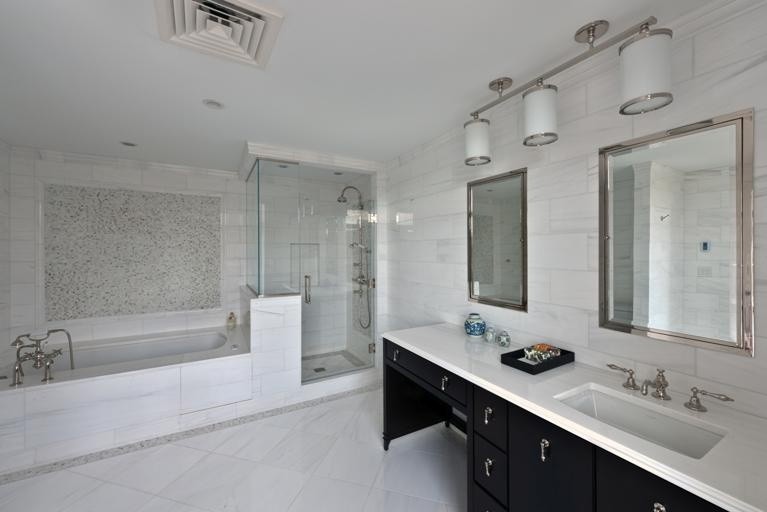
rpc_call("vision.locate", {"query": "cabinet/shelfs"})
[469,386,507,509]
[508,403,594,512]
[595,451,731,512]
[385,341,468,408]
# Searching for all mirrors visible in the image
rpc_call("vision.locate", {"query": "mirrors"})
[596,105,755,358]
[467,159,527,311]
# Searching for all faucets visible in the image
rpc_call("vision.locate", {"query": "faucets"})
[640,368,671,401]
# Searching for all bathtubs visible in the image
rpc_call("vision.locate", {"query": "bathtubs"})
[0,330,251,476]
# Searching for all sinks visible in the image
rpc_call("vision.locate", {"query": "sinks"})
[553,382,729,460]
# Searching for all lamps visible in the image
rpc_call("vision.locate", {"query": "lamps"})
[463,20,677,166]
